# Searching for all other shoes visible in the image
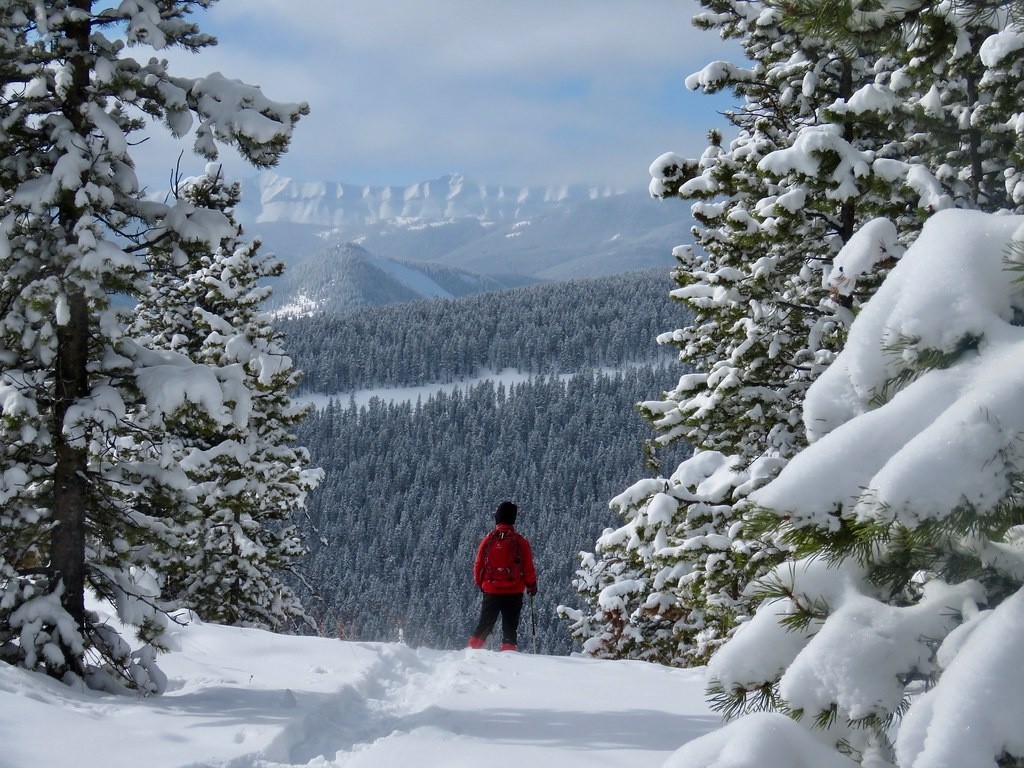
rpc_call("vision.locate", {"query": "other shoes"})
[501,643,518,651]
[469,636,484,649]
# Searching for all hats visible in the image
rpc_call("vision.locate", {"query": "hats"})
[494,501,518,525]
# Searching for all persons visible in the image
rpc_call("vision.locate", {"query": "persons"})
[467,502,538,652]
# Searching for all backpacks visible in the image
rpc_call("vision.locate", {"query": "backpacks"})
[477,532,525,586]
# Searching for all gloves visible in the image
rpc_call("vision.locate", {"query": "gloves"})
[525,582,538,596]
